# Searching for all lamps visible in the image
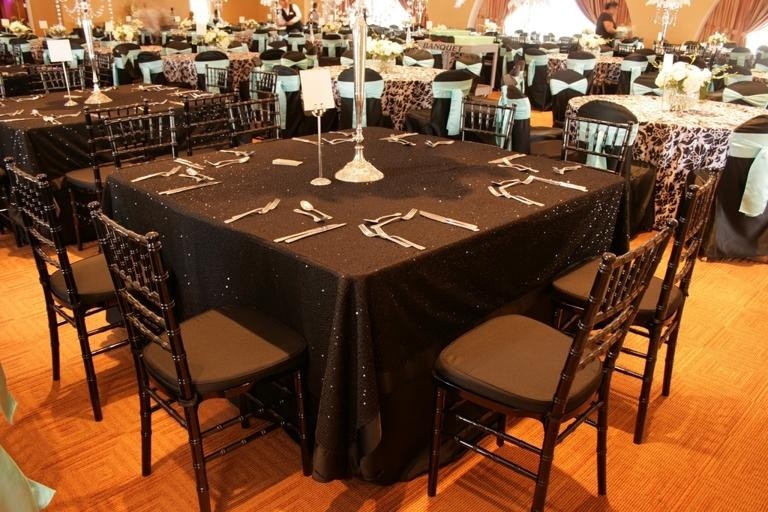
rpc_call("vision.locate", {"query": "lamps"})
[63,0,691,26]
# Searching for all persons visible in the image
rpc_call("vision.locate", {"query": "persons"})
[180,12,196,31]
[307,3,319,25]
[209,9,224,28]
[276,0,303,35]
[596,2,619,48]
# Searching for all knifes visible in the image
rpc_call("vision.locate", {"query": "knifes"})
[420,209,481,232]
[535,176,590,193]
[158,181,221,196]
[173,157,205,171]
[272,222,348,243]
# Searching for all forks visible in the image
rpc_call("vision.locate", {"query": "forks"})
[357,208,426,252]
[503,158,540,172]
[487,174,546,207]
[292,206,328,223]
[131,166,207,183]
[222,198,281,224]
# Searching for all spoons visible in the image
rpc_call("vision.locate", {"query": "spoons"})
[497,164,529,173]
[300,200,333,220]
[186,167,216,180]
[216,156,251,169]
[551,165,581,174]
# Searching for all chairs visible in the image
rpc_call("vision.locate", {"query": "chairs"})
[428,219,679,511]
[557,173,721,443]
[3,17,767,263]
[15,204,130,423]
[84,199,309,512]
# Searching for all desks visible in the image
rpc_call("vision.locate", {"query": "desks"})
[108,124,626,481]
